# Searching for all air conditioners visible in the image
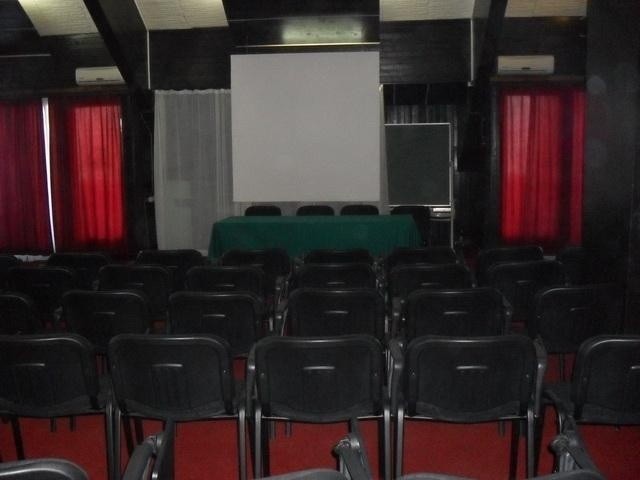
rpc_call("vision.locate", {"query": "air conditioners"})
[74,64,128,87]
[496,55,558,77]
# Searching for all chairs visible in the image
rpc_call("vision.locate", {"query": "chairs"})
[391,206,431,242]
[2,244,636,479]
[296,205,334,216]
[341,204,378,216]
[244,205,281,216]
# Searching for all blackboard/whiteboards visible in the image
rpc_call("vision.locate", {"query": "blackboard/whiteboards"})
[385,122,451,207]
